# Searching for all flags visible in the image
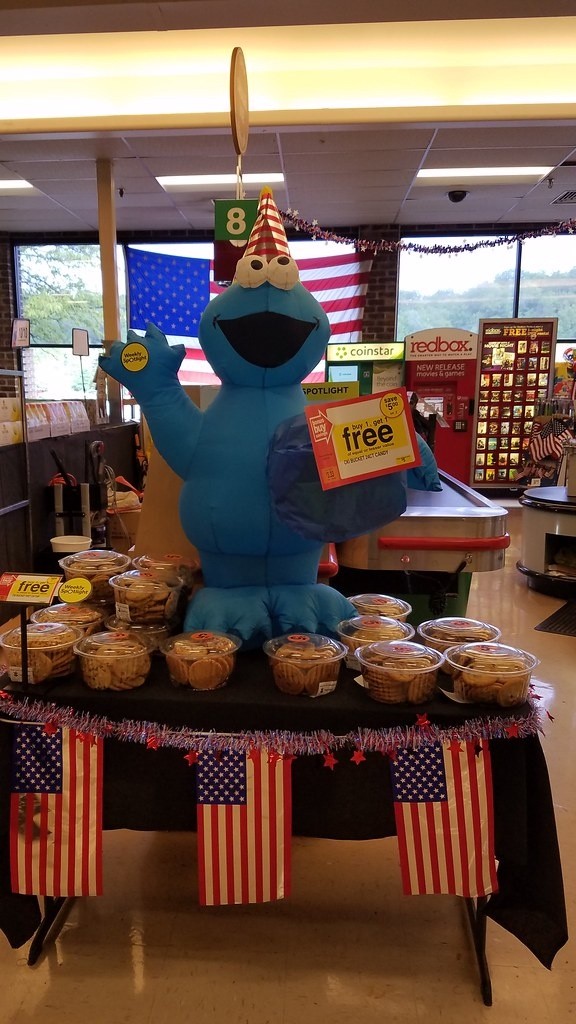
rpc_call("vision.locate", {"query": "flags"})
[529,418,574,459]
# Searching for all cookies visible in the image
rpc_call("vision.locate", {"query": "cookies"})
[3,549,539,708]
